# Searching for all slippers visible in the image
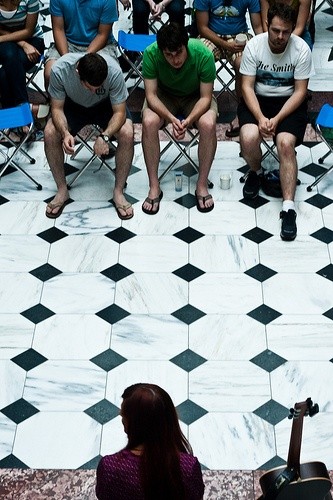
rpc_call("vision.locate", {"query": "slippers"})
[142,191,163,215]
[46,197,70,218]
[194,190,214,213]
[111,197,134,220]
[225,122,240,137]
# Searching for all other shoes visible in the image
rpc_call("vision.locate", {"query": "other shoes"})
[28,103,50,120]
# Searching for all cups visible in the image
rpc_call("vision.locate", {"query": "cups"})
[174,171,184,191]
[220,176,230,190]
[236,34,247,45]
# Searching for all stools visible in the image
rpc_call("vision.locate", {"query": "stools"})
[0,1,332,192]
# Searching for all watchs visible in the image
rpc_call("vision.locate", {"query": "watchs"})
[99,135,110,142]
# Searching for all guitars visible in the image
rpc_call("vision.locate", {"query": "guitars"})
[256,397,332,500]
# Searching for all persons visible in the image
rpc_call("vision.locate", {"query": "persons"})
[43,53,134,220]
[238,3,316,241]
[141,24,218,215]
[95,383,205,500]
[44,0,118,112]
[120,0,313,137]
[0,0,45,143]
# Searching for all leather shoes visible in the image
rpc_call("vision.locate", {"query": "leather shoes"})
[279,209,297,240]
[243,169,265,199]
